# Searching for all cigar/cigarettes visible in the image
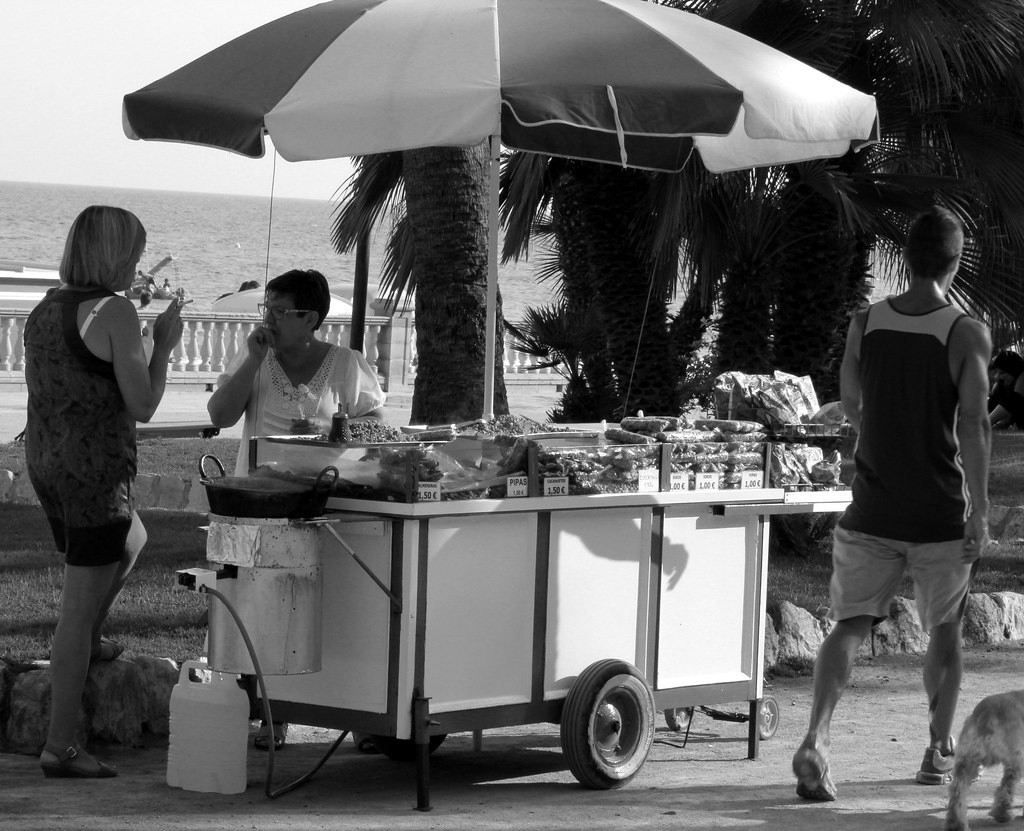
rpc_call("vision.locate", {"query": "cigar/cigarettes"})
[178,300,194,306]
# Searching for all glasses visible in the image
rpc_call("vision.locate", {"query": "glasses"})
[258,303,312,320]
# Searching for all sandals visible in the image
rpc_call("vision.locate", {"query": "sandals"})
[40,743,118,779]
[89,637,124,663]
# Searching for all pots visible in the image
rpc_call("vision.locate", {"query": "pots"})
[198,453,339,520]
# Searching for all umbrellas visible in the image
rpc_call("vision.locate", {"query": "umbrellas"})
[121,0,877,418]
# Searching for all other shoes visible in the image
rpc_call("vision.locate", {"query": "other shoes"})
[256,719,288,750]
[353,731,380,754]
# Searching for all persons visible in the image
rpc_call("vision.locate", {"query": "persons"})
[138,271,158,289]
[792,206,993,805]
[163,278,170,287]
[207,269,386,477]
[25,206,184,778]
[137,290,153,329]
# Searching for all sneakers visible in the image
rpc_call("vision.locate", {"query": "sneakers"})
[917,736,984,785]
[794,730,838,799]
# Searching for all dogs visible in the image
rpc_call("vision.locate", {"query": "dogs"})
[945,690,1024,831]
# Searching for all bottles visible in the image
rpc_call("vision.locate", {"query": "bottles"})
[166,661,250,794]
